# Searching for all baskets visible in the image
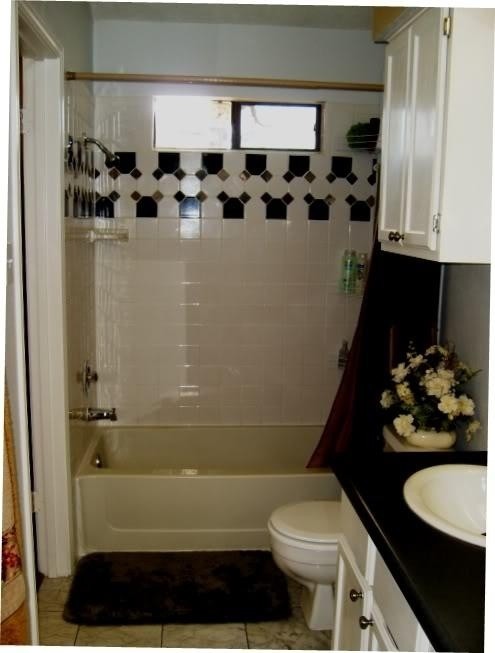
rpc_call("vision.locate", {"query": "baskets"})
[343,133,380,151]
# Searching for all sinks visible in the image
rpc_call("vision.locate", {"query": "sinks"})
[402,464,487,548]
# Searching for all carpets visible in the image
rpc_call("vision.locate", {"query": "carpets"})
[63,551,293,626]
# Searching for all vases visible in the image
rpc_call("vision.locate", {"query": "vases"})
[406,431,457,449]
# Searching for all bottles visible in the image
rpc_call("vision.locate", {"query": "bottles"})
[338,248,358,292]
[356,252,369,289]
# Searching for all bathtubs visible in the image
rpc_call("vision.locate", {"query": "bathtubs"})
[76,425,342,556]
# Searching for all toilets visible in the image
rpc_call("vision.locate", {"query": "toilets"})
[266,425,453,632]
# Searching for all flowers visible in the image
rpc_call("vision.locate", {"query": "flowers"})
[381,340,483,445]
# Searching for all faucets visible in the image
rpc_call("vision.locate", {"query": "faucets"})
[69,408,88,420]
[88,406,118,421]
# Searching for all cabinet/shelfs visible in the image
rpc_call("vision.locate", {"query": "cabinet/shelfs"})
[375,7,494,264]
[330,490,436,652]
